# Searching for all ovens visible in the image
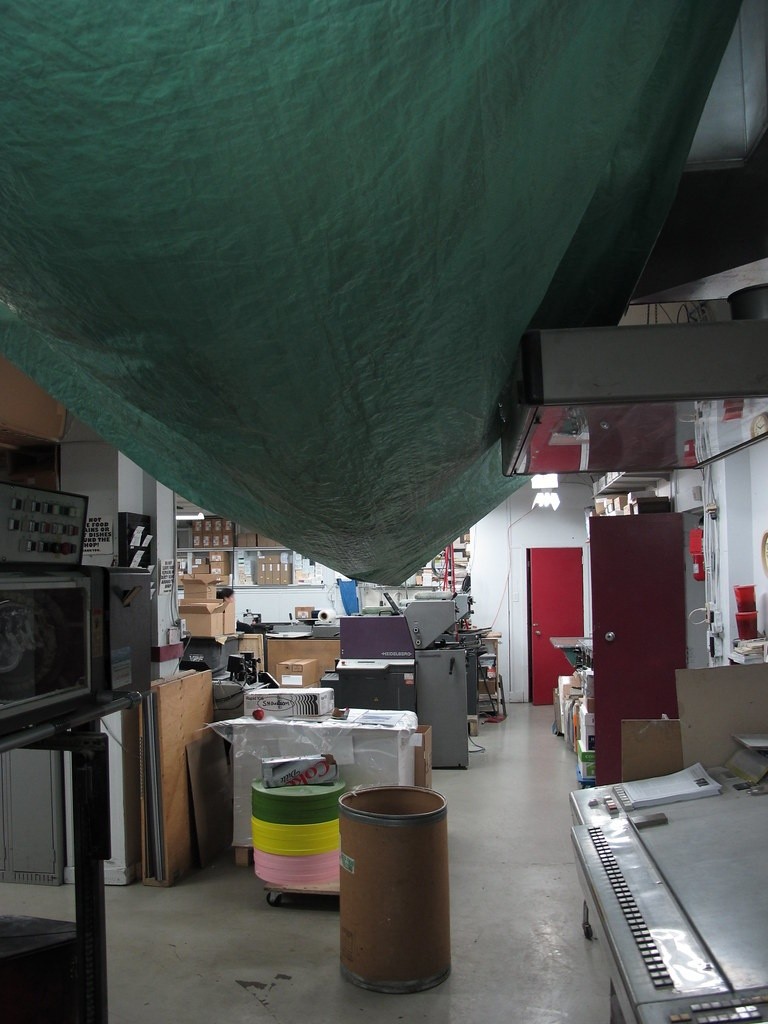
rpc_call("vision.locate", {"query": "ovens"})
[0,573,104,721]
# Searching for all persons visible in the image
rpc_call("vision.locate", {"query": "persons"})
[216,588,235,603]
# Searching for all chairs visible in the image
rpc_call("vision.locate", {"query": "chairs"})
[212,684,244,765]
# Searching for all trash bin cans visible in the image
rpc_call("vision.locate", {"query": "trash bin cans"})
[337,785,451,996]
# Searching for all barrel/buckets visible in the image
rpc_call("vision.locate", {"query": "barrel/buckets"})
[734,583,759,642]
[339,785,451,994]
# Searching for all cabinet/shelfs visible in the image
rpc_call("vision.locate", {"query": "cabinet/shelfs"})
[589,513,685,784]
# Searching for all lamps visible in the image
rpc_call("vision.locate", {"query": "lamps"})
[531,473,561,510]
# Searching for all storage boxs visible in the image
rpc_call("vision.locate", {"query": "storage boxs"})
[223,602,235,634]
[181,574,224,599]
[236,551,291,584]
[585,490,672,544]
[295,607,315,618]
[237,524,284,547]
[244,688,335,716]
[280,681,319,688]
[192,551,231,585]
[276,658,319,687]
[552,670,595,779]
[413,724,431,788]
[193,519,234,546]
[179,599,230,636]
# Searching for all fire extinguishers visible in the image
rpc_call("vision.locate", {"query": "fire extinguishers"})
[691,517,705,581]
